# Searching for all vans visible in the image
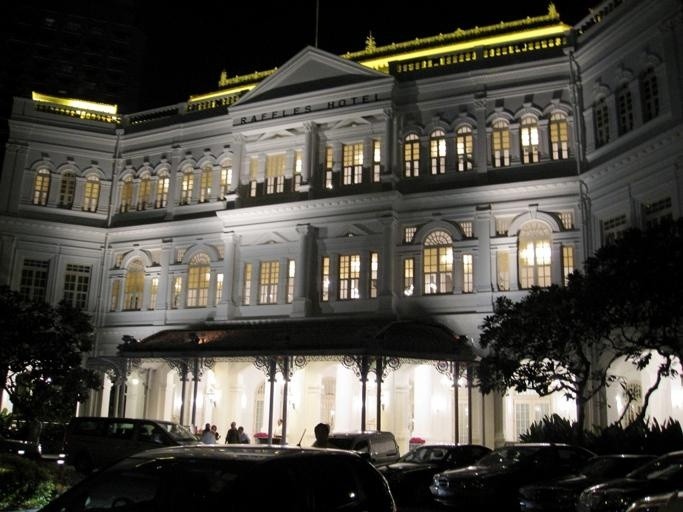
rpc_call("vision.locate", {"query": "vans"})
[1,413,68,462]
[67,414,204,467]
[313,431,399,467]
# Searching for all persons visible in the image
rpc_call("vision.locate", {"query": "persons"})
[237,426,250,443]
[200,423,215,443]
[224,421,241,443]
[273,418,281,436]
[310,422,337,448]
[210,425,220,439]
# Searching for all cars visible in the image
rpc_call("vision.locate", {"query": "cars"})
[428,439,596,509]
[377,444,494,502]
[522,449,683,512]
[37,441,396,512]
